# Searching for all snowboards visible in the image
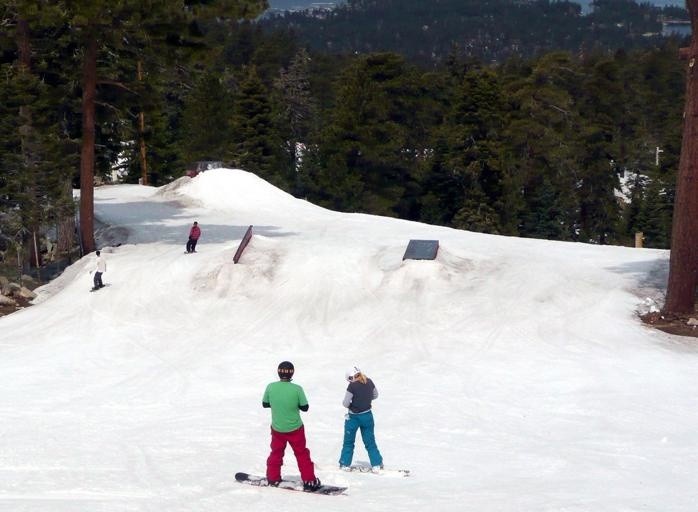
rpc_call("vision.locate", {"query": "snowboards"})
[89,285,105,292]
[184,251,197,254]
[312,462,410,478]
[235,473,347,496]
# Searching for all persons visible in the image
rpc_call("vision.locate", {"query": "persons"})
[90,250,106,290]
[339,365,383,470]
[262,361,321,492]
[186,222,201,253]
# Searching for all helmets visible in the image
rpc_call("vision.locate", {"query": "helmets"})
[278,360,295,379]
[347,364,361,380]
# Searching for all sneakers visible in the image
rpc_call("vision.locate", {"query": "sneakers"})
[266,464,386,492]
